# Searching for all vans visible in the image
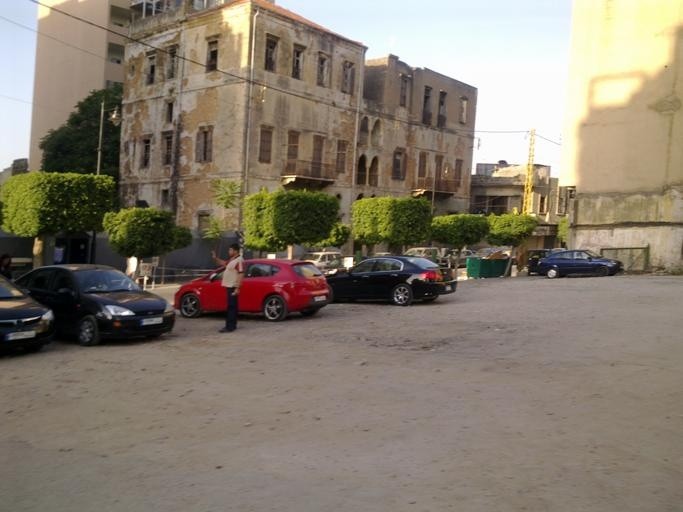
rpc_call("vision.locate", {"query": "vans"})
[295,251,343,271]
[405,247,514,269]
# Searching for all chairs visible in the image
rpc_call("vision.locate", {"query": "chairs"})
[250,267,262,278]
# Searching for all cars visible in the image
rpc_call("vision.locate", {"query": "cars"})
[527,248,621,279]
[325,255,458,306]
[173,259,332,322]
[0,263,176,355]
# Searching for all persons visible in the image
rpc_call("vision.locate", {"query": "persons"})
[0,253,13,281]
[119,250,137,290]
[207,241,245,335]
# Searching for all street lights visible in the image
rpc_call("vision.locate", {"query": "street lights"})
[87,100,124,264]
